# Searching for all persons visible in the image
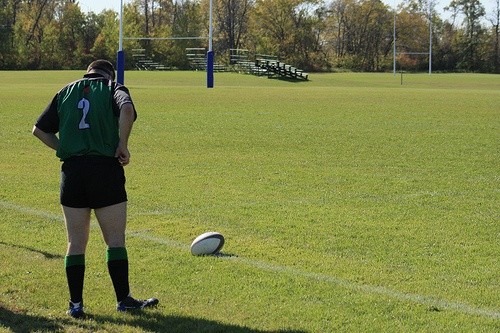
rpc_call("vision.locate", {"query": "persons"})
[31,59,159,318]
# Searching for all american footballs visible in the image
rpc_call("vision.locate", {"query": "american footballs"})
[190,232,225,257]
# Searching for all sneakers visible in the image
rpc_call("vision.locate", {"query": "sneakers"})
[66,299,83,316]
[116,294,159,311]
